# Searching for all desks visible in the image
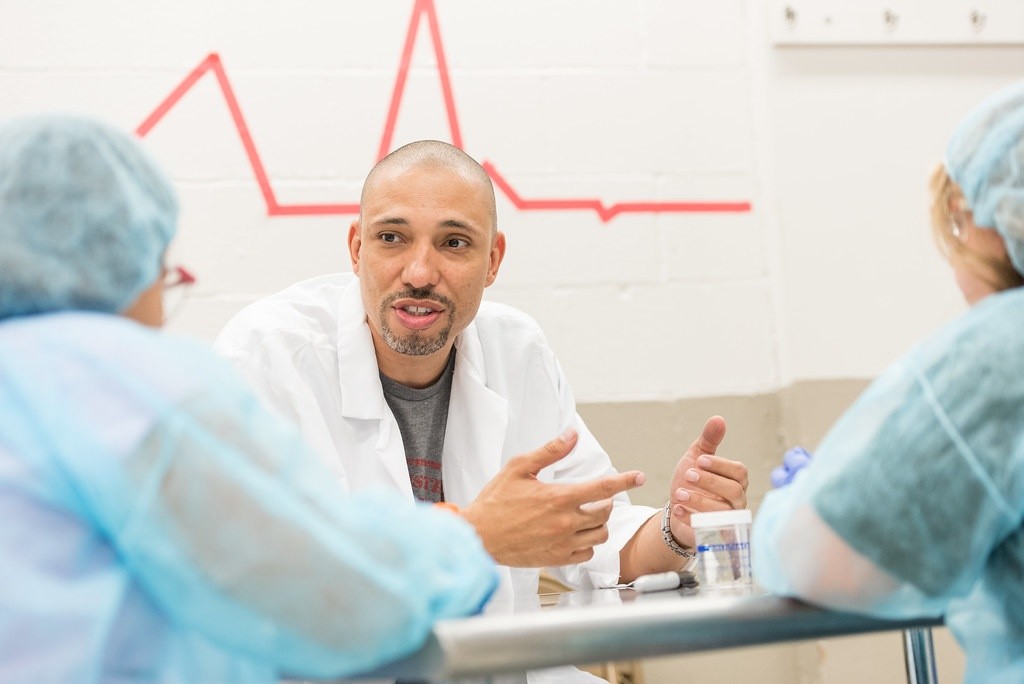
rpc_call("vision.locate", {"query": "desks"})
[432,589,949,683]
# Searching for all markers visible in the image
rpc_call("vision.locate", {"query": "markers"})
[631,570,698,593]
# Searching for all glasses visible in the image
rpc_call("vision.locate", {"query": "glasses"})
[158,266,194,289]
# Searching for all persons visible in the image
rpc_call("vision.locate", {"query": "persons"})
[0,102,501,684]
[205,136,750,684]
[746,81,1022,684]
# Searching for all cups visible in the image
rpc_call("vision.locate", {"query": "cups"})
[690,508,752,587]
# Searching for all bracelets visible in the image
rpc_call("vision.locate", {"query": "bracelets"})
[661,499,698,566]
